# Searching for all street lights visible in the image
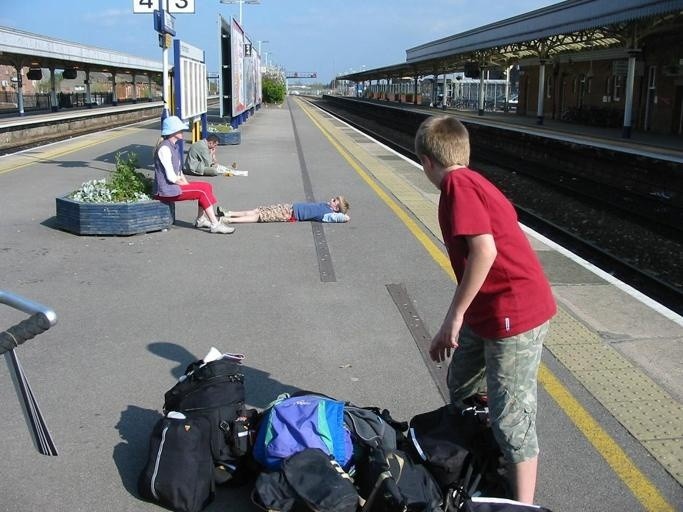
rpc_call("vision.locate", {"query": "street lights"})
[337,65,366,95]
[222,0,256,24]
[255,40,268,55]
[263,51,272,71]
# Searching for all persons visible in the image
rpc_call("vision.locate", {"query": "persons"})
[217,196,351,224]
[183,133,219,178]
[153,115,236,234]
[415,115,558,505]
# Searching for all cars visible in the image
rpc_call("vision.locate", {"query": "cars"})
[485,93,517,111]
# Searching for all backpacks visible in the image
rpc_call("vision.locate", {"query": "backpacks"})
[407,393,500,496]
[253,390,409,486]
[163,357,252,497]
[356,448,444,511]
[249,447,367,510]
[137,410,233,512]
[460,497,548,511]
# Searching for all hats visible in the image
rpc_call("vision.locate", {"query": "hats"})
[160,115,189,136]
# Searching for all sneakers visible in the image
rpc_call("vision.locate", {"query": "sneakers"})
[195,207,235,234]
